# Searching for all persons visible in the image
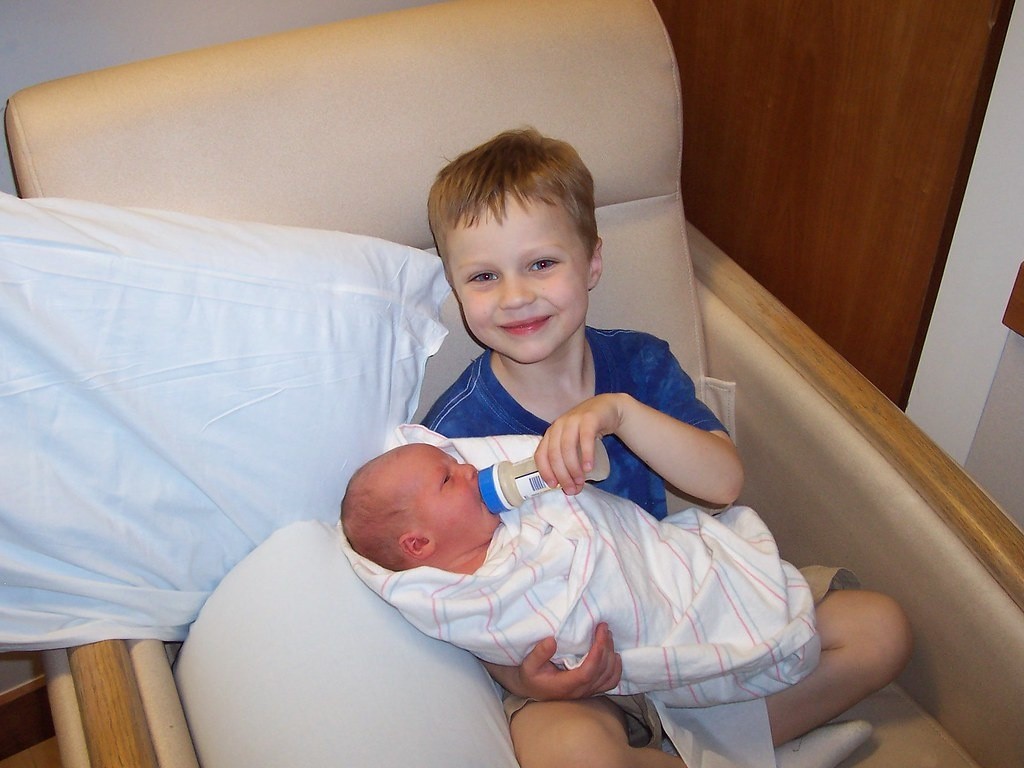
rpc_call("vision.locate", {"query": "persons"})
[340,443,820,709]
[419,128,911,768]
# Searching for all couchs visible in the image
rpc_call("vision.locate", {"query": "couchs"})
[3,2,1024,768]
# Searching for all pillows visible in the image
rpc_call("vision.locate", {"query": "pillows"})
[170,516,522,768]
[0,191,450,652]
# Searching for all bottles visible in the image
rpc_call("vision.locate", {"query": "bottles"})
[477,434,610,514]
